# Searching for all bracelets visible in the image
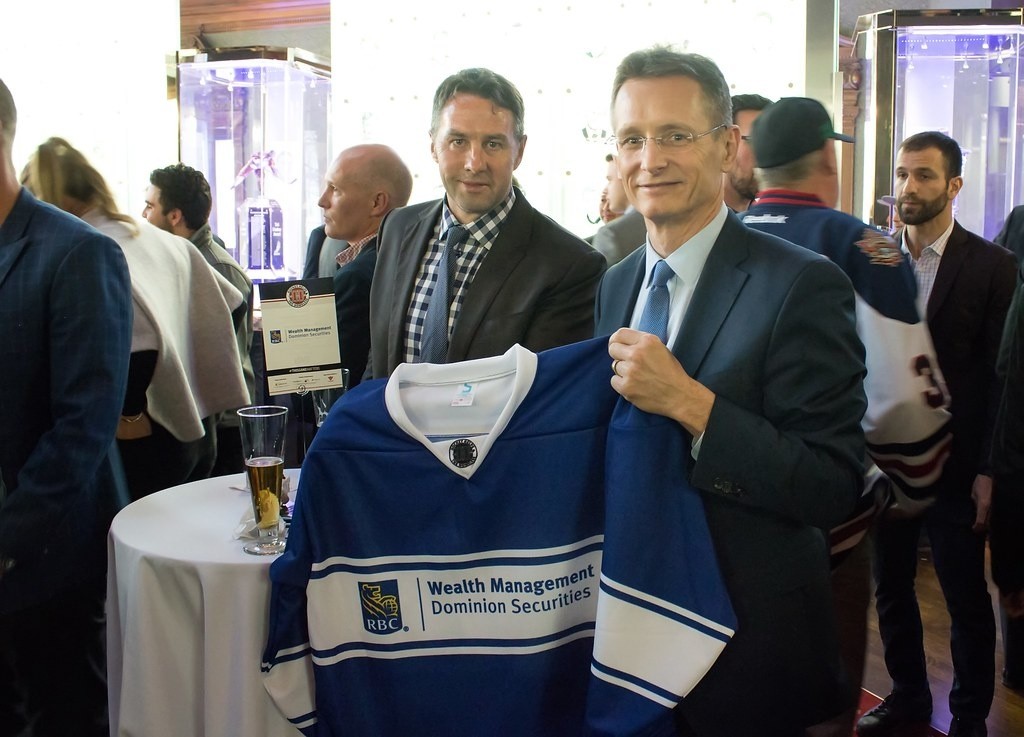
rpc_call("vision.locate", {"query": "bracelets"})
[121,412,143,423]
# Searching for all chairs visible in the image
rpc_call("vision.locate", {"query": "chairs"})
[110,349,187,510]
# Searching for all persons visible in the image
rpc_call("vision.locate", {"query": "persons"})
[993,205,1024,282]
[370,67,608,380]
[857,131,1018,737]
[882,196,906,231]
[970,283,1024,697]
[592,43,869,737]
[291,144,413,468]
[0,80,134,737]
[592,153,647,269]
[20,137,251,501]
[735,97,954,737]
[582,187,624,246]
[142,162,253,478]
[724,93,774,214]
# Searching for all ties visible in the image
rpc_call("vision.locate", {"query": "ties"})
[638,260,675,346]
[419,225,469,364]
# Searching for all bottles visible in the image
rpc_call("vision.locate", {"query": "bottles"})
[236,153,284,277]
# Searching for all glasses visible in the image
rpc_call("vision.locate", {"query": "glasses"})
[616,123,727,153]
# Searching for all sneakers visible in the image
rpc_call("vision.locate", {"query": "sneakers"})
[856,687,933,737]
[948,718,988,737]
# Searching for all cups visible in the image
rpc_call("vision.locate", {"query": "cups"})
[236,405,290,555]
[312,369,348,427]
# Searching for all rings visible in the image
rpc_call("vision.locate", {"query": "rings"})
[613,360,622,377]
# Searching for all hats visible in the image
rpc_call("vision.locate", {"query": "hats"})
[751,97,856,168]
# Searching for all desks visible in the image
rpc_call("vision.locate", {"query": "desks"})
[107,468,307,736]
